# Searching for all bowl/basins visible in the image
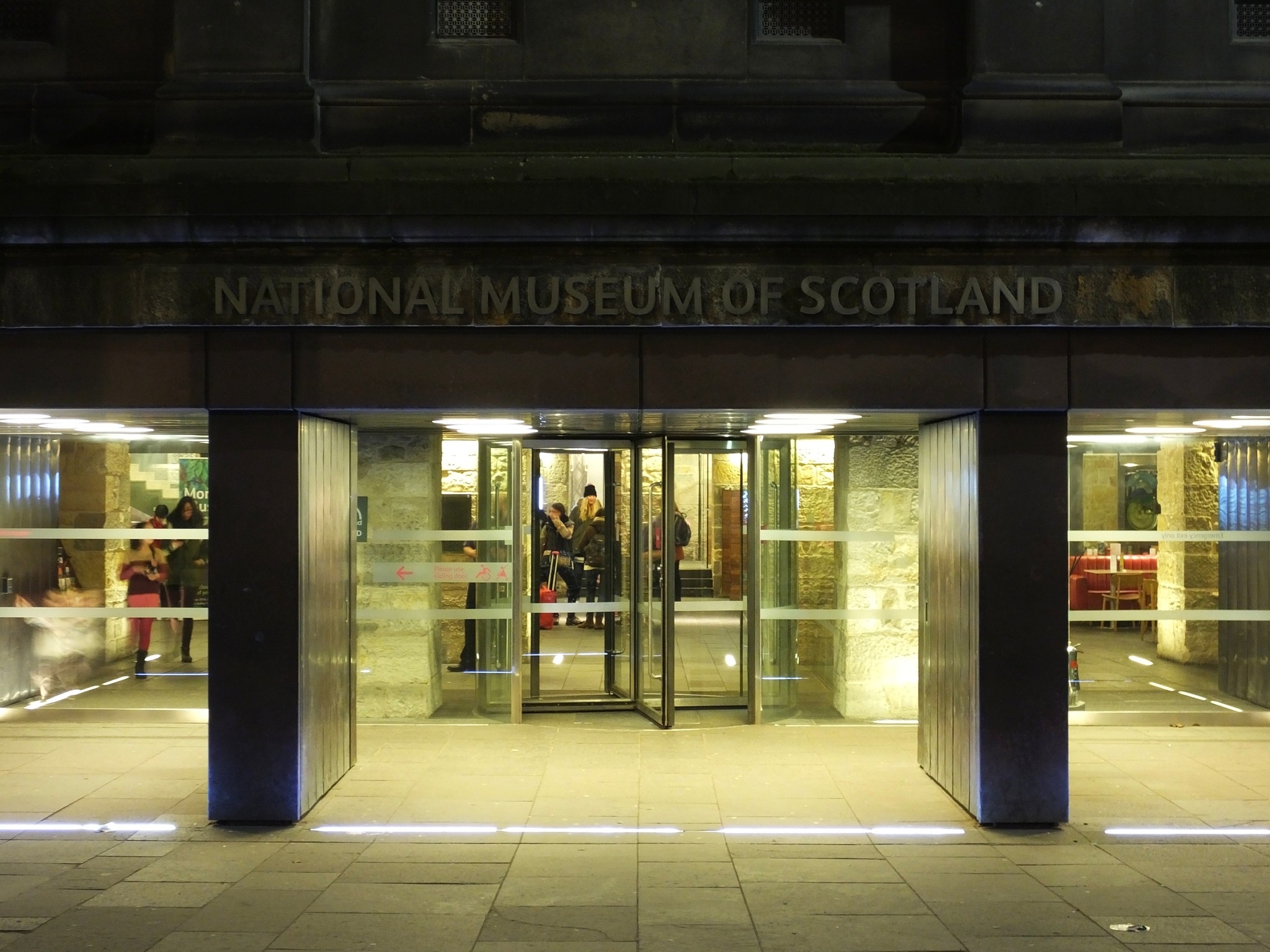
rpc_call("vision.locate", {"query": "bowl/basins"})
[1087,549,1098,555]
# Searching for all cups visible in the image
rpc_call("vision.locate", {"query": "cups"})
[1150,548,1155,556]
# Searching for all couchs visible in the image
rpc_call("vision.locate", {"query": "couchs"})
[1069,553,1157,611]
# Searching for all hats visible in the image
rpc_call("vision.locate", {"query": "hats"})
[584,485,597,497]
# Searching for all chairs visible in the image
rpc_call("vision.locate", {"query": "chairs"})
[1101,572,1157,643]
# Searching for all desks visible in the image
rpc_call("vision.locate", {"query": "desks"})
[1082,566,1156,630]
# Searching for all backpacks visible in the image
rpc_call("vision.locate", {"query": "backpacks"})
[585,523,605,567]
[674,512,691,546]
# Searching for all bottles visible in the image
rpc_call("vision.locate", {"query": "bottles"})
[1128,544,1132,555]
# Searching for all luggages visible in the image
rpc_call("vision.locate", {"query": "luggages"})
[539,551,559,630]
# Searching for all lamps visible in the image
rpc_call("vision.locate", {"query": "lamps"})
[1214,438,1227,462]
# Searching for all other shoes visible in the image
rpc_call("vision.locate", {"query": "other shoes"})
[565,616,585,625]
[553,617,559,625]
[447,660,476,673]
[615,619,622,625]
[135,650,148,679]
[180,643,192,663]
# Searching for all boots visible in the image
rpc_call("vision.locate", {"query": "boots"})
[578,614,594,629]
[594,615,605,629]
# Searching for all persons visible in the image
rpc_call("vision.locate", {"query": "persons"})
[160,496,208,663]
[120,522,168,680]
[146,505,178,621]
[674,500,691,603]
[447,494,509,678]
[539,485,605,629]
